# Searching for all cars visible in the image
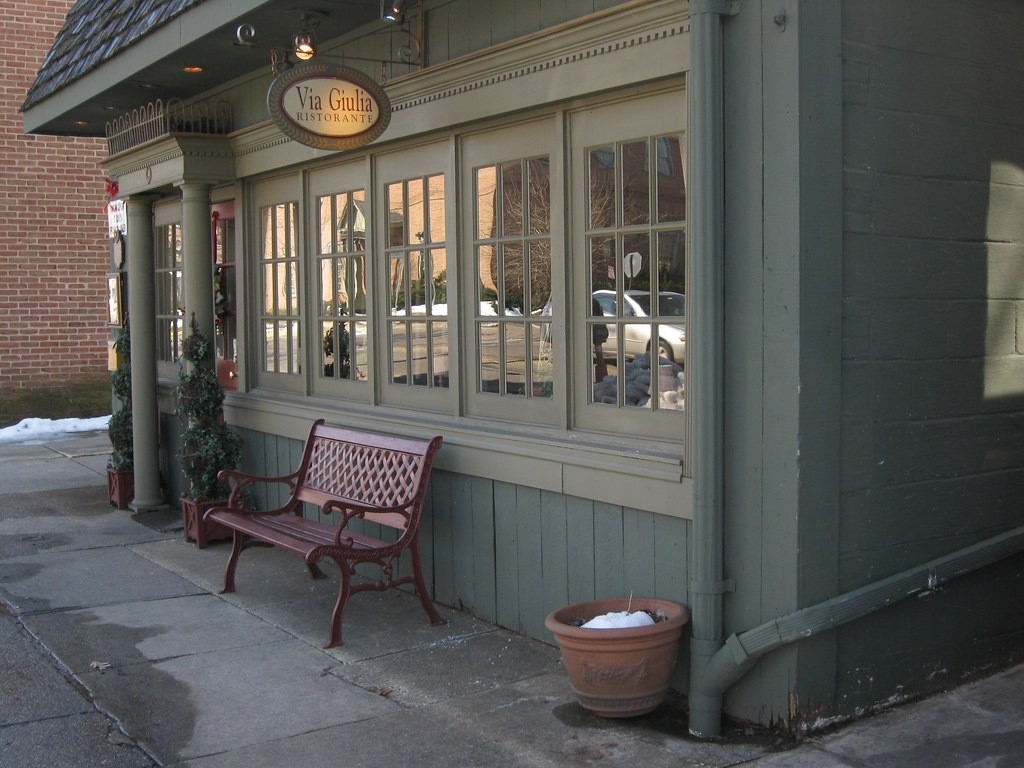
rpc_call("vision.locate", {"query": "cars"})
[539,289,685,365]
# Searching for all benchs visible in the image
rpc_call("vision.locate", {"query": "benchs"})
[202,419,448,649]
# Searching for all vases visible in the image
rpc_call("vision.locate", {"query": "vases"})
[545,599,688,717]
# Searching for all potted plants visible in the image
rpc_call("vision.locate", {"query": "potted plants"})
[105,311,162,509]
[178,311,243,549]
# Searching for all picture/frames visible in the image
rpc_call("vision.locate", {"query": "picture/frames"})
[105,273,122,328]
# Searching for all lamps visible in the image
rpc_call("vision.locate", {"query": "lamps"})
[295,9,319,59]
